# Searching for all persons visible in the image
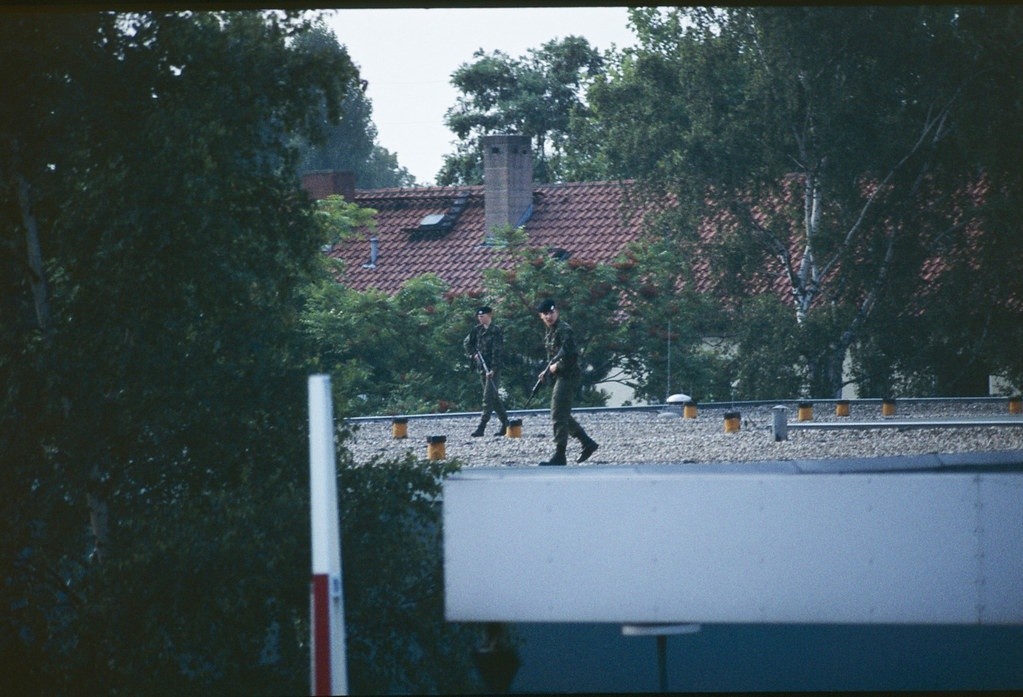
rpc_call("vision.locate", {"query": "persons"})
[463,306,509,437]
[537,299,599,466]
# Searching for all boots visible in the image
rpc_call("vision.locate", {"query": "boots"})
[471,426,485,436]
[538,446,567,465]
[576,430,598,463]
[494,423,507,435]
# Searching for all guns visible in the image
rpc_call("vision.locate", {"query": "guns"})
[522,347,563,410]
[476,348,499,393]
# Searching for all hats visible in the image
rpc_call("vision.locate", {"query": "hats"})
[537,300,555,312]
[476,307,492,315]
